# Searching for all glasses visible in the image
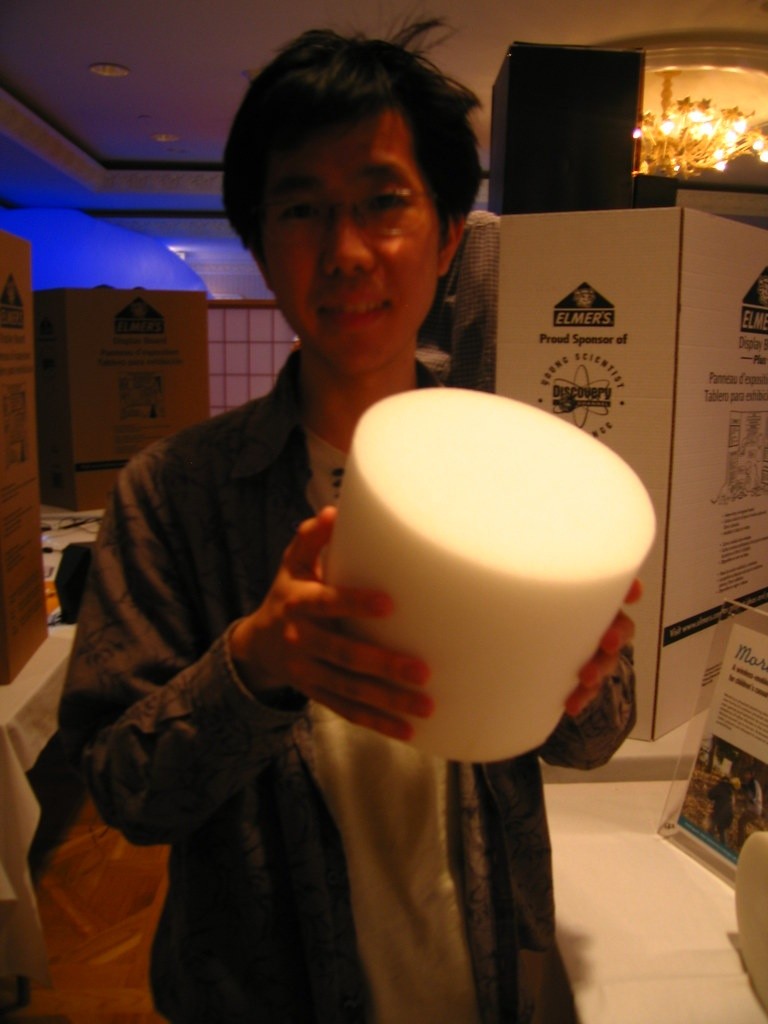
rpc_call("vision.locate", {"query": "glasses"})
[259,189,431,247]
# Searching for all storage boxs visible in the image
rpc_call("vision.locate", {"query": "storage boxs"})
[35,286,211,513]
[493,206,767,741]
[0,228,47,685]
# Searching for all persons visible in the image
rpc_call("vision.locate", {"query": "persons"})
[709,770,762,847]
[56,28,644,1024]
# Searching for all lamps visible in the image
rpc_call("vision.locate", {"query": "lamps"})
[600,31,768,181]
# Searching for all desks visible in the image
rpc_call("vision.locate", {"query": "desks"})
[0,505,104,1008]
